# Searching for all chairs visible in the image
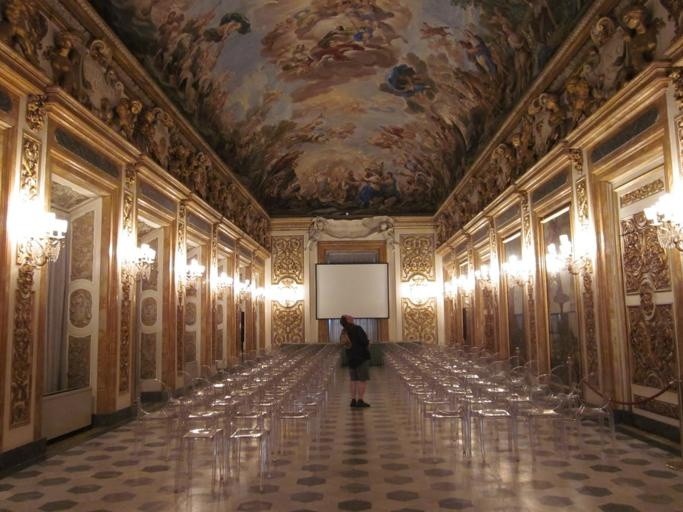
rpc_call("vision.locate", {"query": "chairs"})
[381,341,621,461]
[134,341,344,492]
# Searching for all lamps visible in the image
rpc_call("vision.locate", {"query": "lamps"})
[442,191,682,302]
[14,210,264,305]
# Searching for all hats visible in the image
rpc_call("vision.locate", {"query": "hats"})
[339,332,352,350]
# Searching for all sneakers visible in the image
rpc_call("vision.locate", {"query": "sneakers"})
[350,401,370,407]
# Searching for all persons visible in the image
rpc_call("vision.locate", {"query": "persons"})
[340,315,375,407]
[2,0,268,246]
[435,1,683,247]
